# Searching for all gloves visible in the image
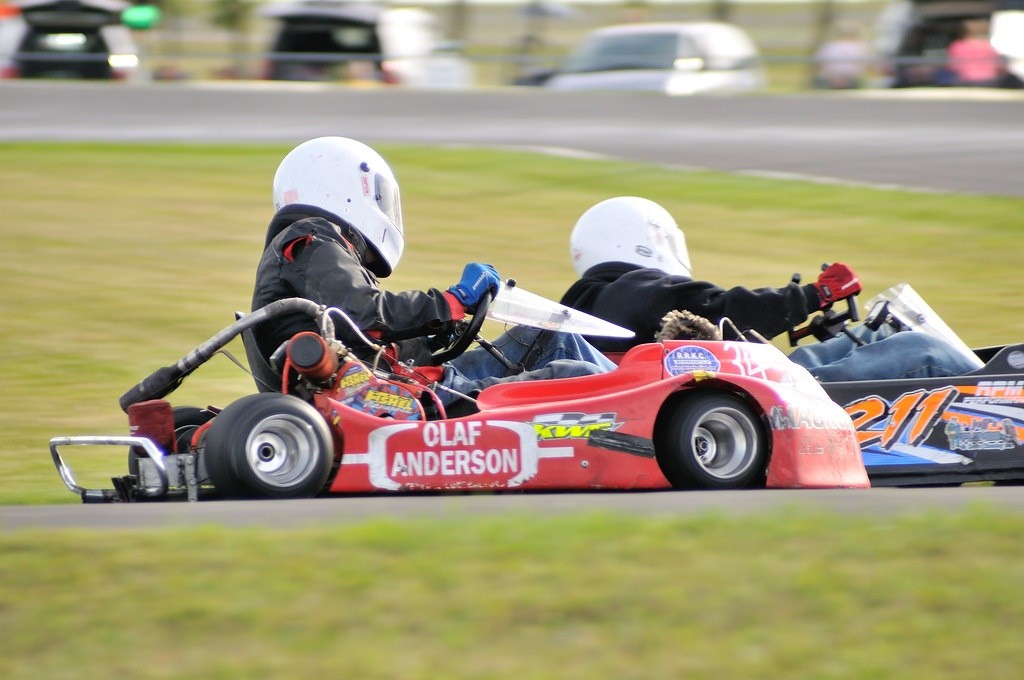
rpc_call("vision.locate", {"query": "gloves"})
[812,263,861,313]
[445,262,501,305]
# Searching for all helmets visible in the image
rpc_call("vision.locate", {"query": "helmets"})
[273,136,405,277]
[570,196,692,279]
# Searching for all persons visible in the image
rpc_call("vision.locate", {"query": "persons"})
[815,18,1007,88]
[249,136,619,419]
[558,196,980,381]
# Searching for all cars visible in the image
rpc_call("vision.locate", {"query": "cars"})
[1,0,165,82]
[874,0,1024,90]
[538,20,764,95]
[249,5,480,89]
